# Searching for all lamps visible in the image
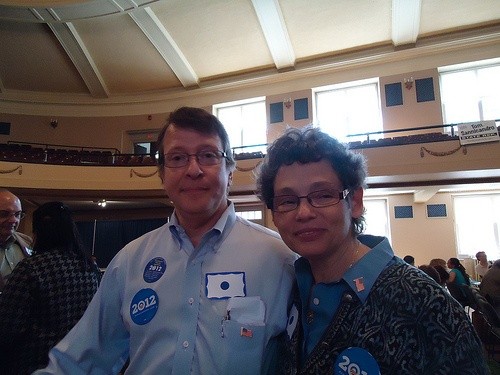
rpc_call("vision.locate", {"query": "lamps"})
[283,98,292,108]
[403,77,413,89]
[50,118,58,127]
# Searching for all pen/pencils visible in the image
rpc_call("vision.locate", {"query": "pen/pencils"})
[221,309,230,338]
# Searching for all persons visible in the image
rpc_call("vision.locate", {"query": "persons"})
[403,254,470,310]
[256,128,488,375]
[33,105,299,375]
[0,188,33,293]
[475,252,493,281]
[0,201,101,375]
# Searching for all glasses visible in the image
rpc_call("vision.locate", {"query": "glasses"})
[0,209,25,219]
[266,187,352,212]
[156,149,227,168]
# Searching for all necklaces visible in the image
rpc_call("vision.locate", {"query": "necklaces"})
[354,242,361,262]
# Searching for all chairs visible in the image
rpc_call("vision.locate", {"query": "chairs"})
[0,143,114,167]
